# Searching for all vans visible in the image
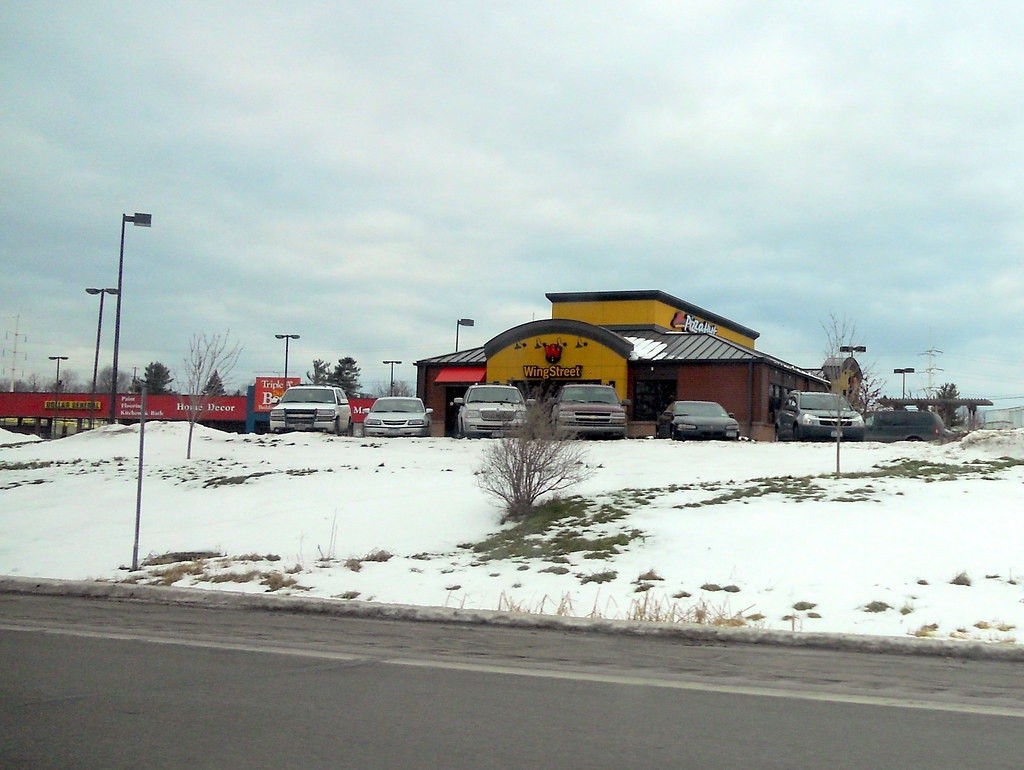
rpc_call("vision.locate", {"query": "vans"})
[862,411,945,443]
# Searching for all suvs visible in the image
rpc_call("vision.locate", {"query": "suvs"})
[550,385,631,438]
[269,383,355,437]
[454,383,538,439]
[774,390,864,442]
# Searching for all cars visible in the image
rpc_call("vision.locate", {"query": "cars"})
[655,401,740,442]
[362,396,434,437]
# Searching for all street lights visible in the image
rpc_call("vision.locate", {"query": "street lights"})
[382,360,401,397]
[275,334,300,392]
[454,318,474,352]
[839,344,867,359]
[50,355,67,439]
[109,212,153,424]
[894,367,914,400]
[84,287,119,429]
